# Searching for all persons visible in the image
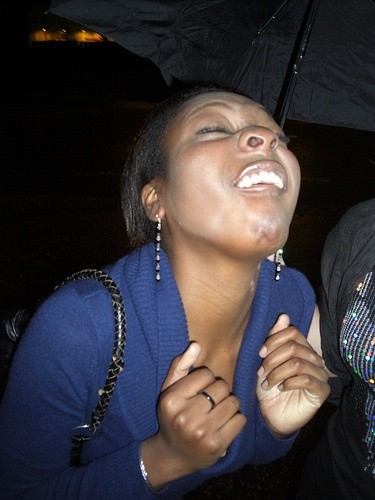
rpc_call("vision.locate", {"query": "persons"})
[0,87,331,498]
[300,196,374,500]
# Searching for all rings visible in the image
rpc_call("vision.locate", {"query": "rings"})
[196,389,216,411]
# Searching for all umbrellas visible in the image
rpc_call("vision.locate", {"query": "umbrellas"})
[17,0,375,133]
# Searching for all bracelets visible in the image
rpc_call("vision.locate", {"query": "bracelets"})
[137,439,158,495]
